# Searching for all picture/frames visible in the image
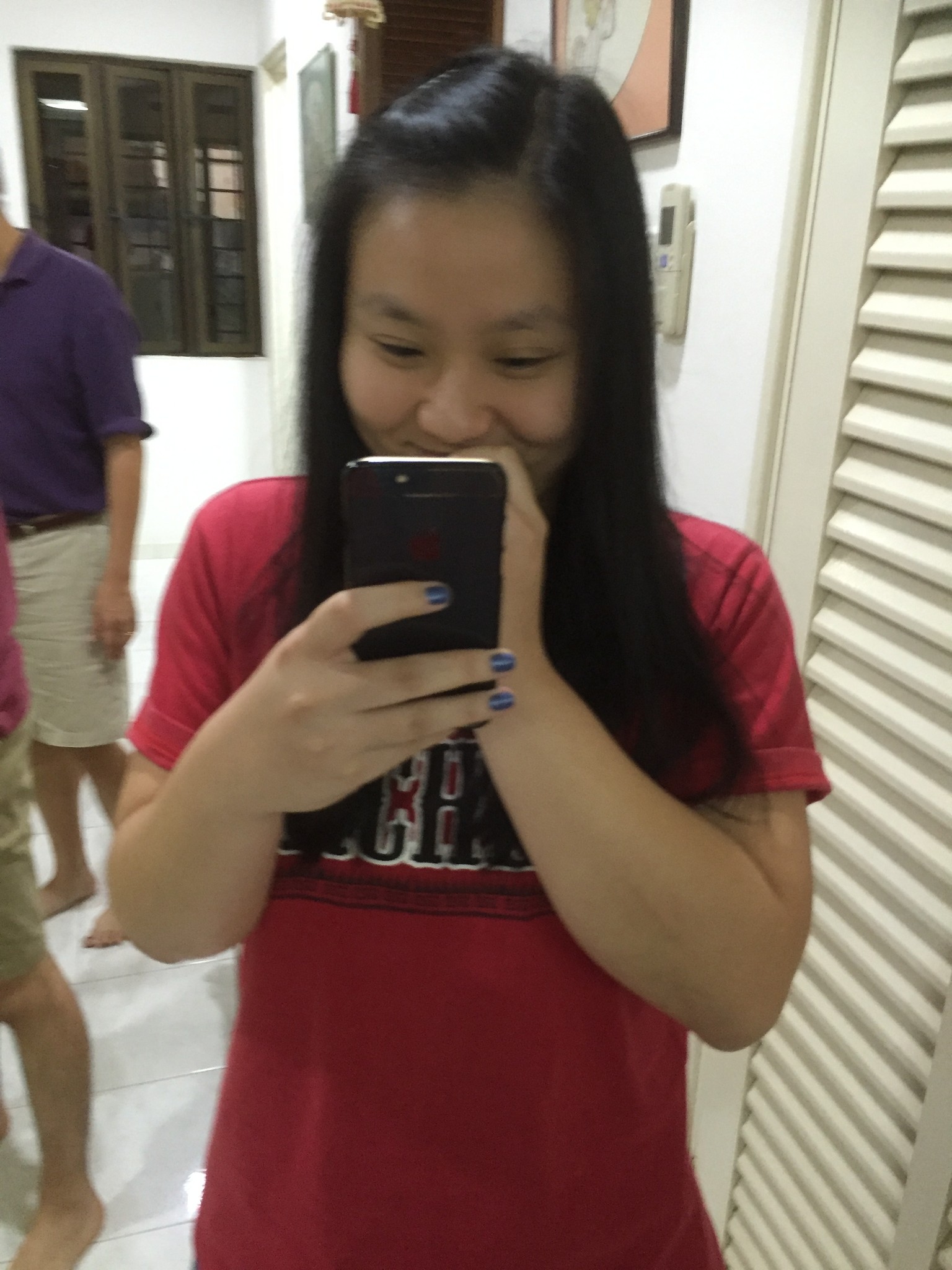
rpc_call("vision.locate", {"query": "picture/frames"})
[548,0,693,157]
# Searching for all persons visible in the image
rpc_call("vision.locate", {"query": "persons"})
[101,43,835,1270]
[0,156,155,1270]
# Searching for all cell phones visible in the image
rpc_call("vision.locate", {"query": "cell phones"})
[342,454,506,729]
